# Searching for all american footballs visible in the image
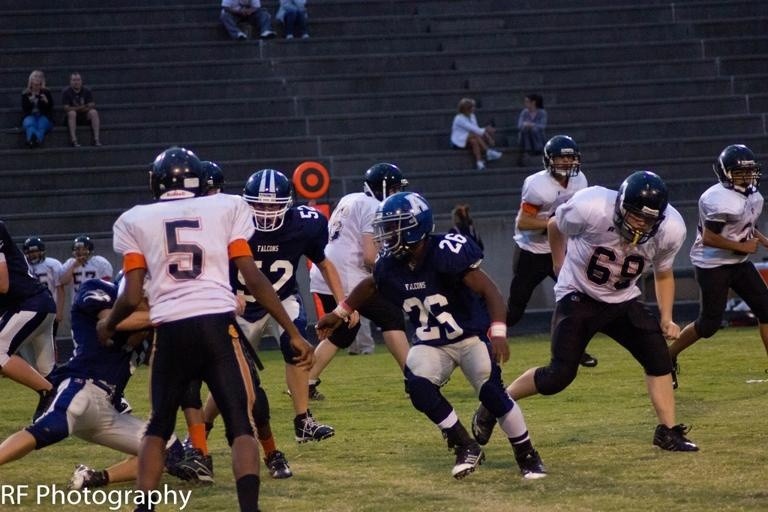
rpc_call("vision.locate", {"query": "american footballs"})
[124,298,150,346]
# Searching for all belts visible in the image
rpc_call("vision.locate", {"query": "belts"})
[92,381,115,397]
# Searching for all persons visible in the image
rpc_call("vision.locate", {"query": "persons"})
[276,0,312,41]
[60,70,103,148]
[19,68,54,149]
[515,92,550,157]
[217,0,277,43]
[662,143,768,389]
[446,97,502,172]
[470,169,699,454]
[504,134,597,369]
[0,145,545,512]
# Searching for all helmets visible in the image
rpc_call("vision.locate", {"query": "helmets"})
[613,170,668,246]
[23,238,44,253]
[242,170,292,232]
[362,164,434,258]
[147,145,225,200]
[72,236,95,258]
[541,133,580,177]
[713,144,761,196]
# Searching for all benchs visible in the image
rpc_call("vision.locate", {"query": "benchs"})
[0,0,768,243]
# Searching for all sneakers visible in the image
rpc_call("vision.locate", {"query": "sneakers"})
[66,463,95,490]
[308,376,324,400]
[294,409,335,443]
[33,387,57,422]
[581,351,598,367]
[264,450,292,478]
[236,31,249,39]
[652,423,701,451]
[488,149,502,161]
[262,30,276,38]
[453,401,550,480]
[176,436,215,486]
[477,160,486,169]
